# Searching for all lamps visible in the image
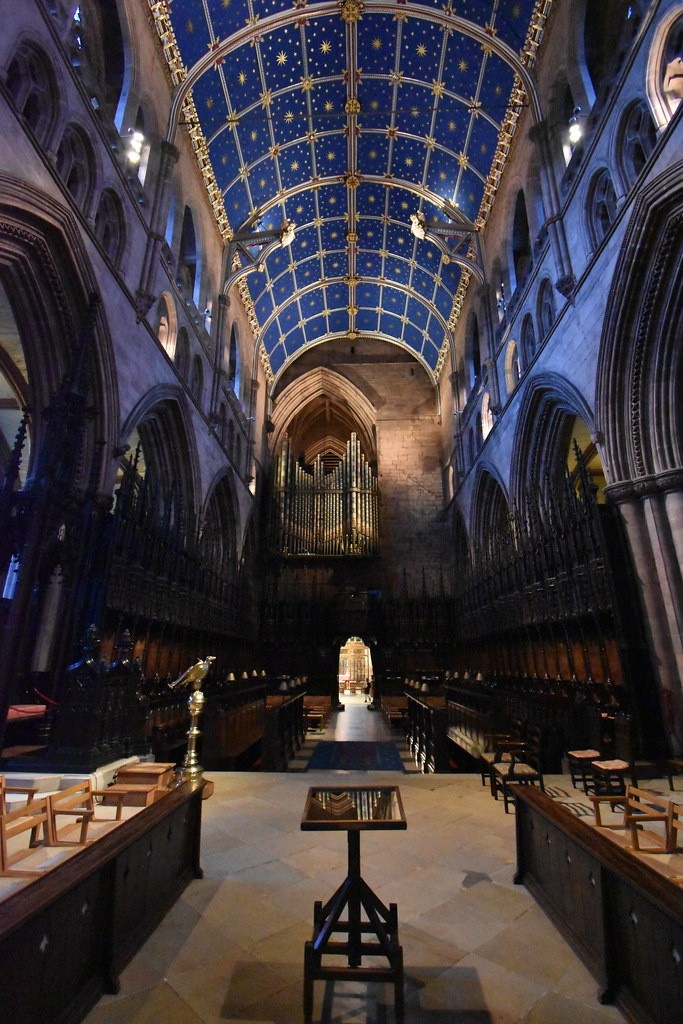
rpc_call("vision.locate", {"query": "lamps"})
[404,678,430,704]
[474,672,484,684]
[444,671,452,681]
[250,669,258,677]
[453,672,461,680]
[260,670,267,678]
[464,672,470,683]
[280,677,307,703]
[219,672,235,687]
[236,671,248,679]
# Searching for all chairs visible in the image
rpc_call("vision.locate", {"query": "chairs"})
[476,717,546,812]
[566,693,637,802]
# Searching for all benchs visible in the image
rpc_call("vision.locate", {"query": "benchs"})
[291,701,331,730]
[380,695,448,730]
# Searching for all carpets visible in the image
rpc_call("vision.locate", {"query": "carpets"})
[305,741,405,771]
